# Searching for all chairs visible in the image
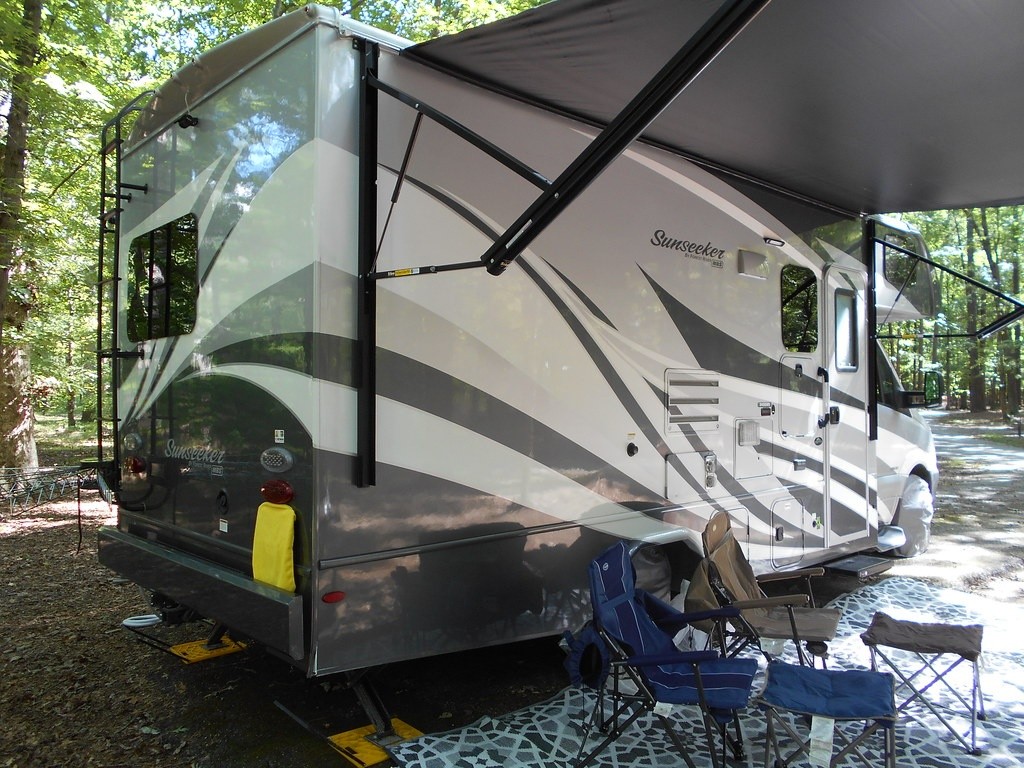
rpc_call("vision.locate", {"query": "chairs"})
[573,541,759,768]
[685,509,842,669]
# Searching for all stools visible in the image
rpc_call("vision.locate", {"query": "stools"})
[754,660,897,768]
[860,611,987,756]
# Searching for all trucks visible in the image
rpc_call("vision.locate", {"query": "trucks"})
[85,5,940,688]
